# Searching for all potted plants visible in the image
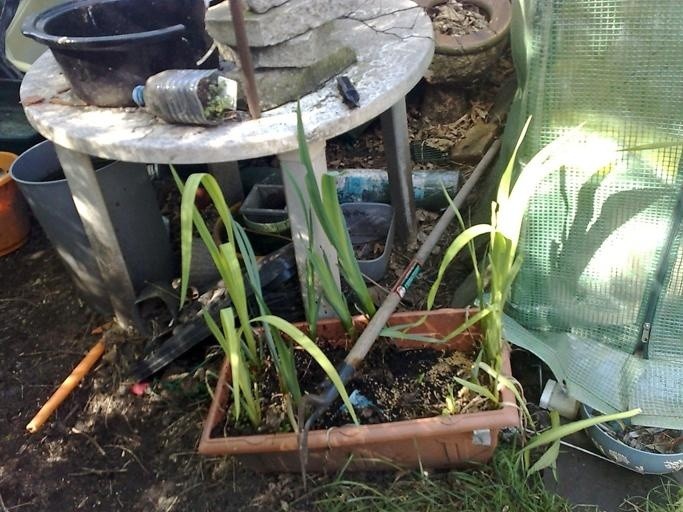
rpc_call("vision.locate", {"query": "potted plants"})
[168,97,518,477]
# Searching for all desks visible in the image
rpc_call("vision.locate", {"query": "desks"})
[20,0,436,329]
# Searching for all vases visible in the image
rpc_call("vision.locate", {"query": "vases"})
[21,0,219,106]
[0,152,30,256]
[416,0,513,126]
[214,202,300,314]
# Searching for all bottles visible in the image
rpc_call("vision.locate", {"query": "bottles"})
[538,378,582,420]
[132,66,238,127]
